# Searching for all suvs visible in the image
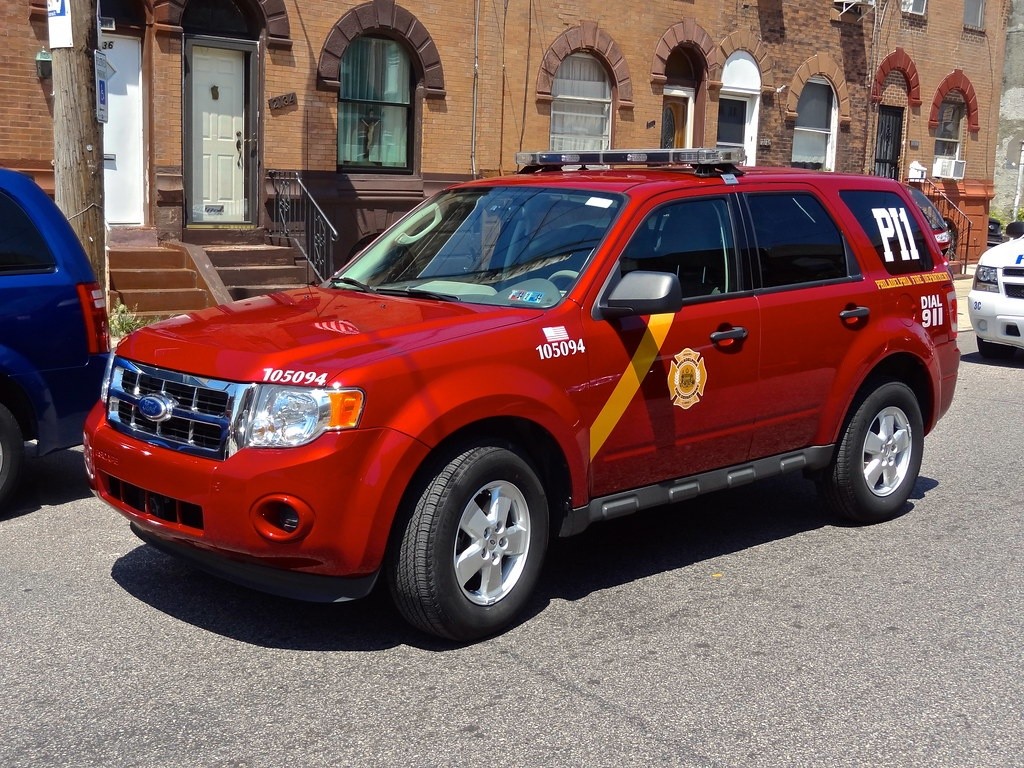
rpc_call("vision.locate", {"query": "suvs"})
[80,144,961,652]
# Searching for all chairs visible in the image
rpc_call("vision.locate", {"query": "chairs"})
[513,200,648,296]
[658,213,733,296]
[744,210,846,289]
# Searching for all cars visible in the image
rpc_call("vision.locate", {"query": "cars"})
[1,167,111,504]
[965,221,1024,356]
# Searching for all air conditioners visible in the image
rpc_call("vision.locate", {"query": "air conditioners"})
[933,158,966,180]
[900,0,927,15]
